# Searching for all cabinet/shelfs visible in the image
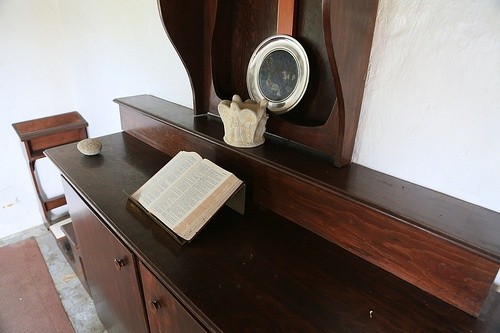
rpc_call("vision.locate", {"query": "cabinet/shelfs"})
[40,91,499,333]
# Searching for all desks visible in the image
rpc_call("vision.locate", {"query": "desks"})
[12,111,89,232]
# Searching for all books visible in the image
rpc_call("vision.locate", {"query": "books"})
[129,150,248,246]
